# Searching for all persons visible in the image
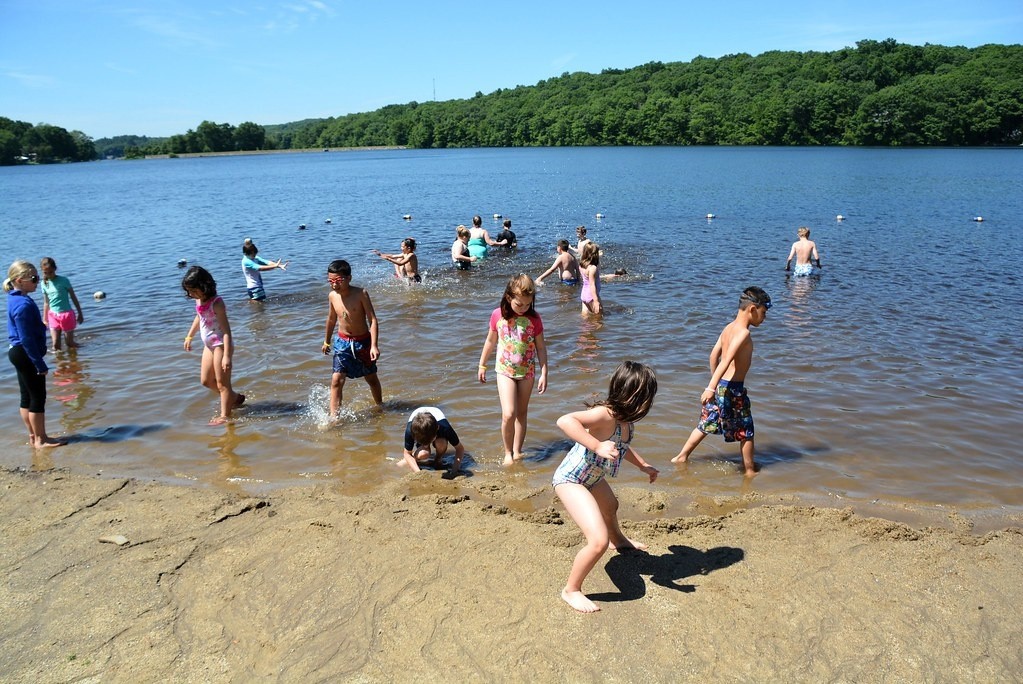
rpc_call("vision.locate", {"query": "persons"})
[552,360,660,613]
[452,225,477,270]
[396,407,464,473]
[478,273,548,465]
[242,244,289,303]
[3,261,68,449]
[670,286,773,477]
[533,224,655,288]
[371,237,423,285]
[317,260,383,431]
[494,219,518,248]
[579,242,604,319]
[40,257,84,350]
[181,266,245,427]
[465,216,508,260]
[786,226,822,277]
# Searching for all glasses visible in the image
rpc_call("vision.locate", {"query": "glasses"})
[21,276,40,283]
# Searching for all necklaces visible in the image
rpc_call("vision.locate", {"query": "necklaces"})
[339,300,353,319]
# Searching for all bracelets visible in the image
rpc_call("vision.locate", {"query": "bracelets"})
[324,341,331,347]
[184,337,194,340]
[705,388,715,393]
[478,365,487,370]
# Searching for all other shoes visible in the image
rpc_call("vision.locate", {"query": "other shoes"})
[208,416,227,426]
[235,394,245,407]
